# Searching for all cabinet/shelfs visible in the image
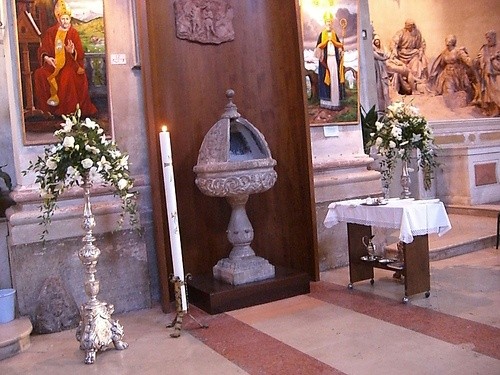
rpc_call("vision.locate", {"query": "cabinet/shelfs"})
[324,198,452,308]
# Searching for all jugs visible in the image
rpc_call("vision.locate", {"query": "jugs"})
[362,235,377,258]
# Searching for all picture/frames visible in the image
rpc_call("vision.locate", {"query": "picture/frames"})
[297,0,362,127]
[11,0,114,146]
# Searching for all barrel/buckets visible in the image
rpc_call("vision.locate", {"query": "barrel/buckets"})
[0,289,16,323]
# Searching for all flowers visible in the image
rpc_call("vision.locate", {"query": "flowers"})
[21,103,147,252]
[358,96,445,199]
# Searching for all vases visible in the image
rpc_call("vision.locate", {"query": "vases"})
[75,171,129,366]
[393,150,420,281]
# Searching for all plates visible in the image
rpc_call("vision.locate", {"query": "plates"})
[360,202,388,206]
[360,255,382,261]
[387,262,403,269]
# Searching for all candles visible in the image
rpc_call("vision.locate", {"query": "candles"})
[158,126,187,312]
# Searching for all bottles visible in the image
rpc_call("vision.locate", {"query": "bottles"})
[366,195,372,204]
